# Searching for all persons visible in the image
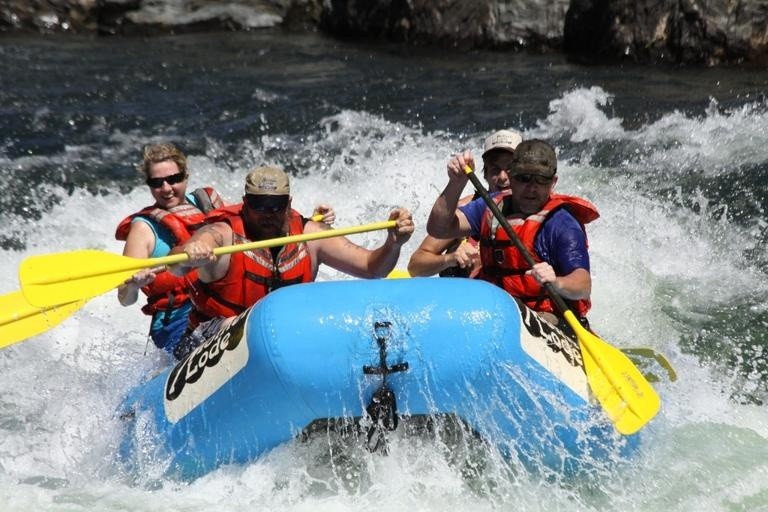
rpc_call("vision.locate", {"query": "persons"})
[425,137,594,348]
[406,129,522,278]
[117,144,337,360]
[165,165,417,359]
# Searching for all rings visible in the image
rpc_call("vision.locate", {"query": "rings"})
[193,246,200,251]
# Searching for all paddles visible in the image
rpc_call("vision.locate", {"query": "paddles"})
[463,165,662,434]
[619,346,677,382]
[0,215,324,348]
[19,221,397,308]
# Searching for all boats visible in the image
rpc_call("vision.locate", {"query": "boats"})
[103,275,642,493]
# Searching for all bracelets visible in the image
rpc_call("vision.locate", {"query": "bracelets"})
[443,255,451,268]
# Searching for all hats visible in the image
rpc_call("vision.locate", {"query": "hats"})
[511,139,557,178]
[245,167,290,199]
[482,129,522,157]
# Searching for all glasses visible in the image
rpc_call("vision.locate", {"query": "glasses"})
[251,205,285,213]
[514,173,552,185]
[146,171,185,188]
